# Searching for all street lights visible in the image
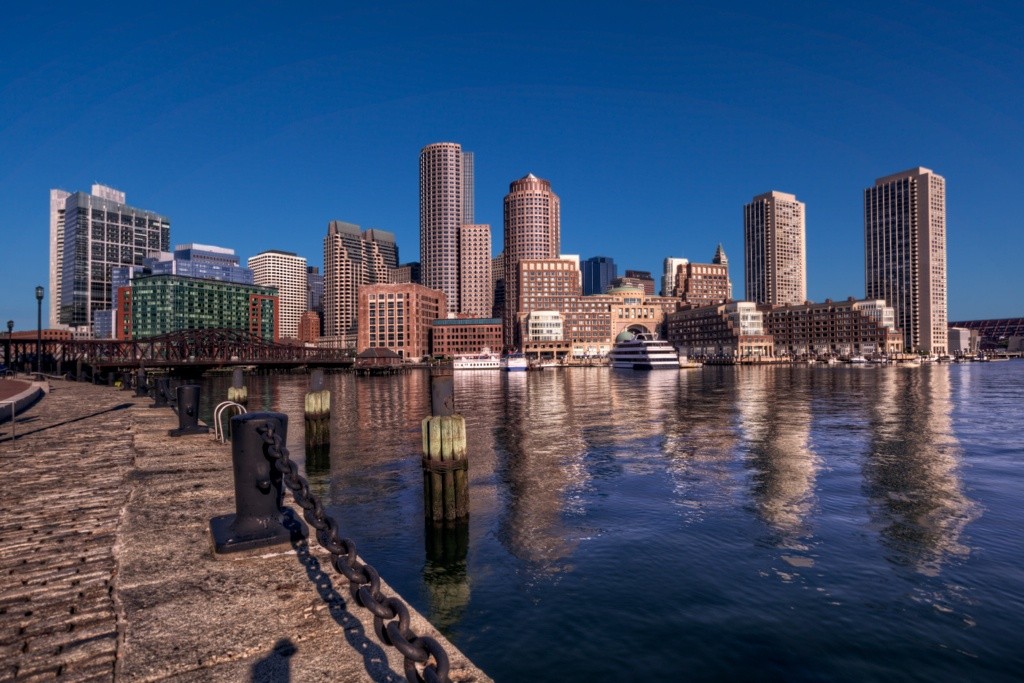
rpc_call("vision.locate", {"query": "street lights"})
[7,320,14,376]
[33,286,45,381]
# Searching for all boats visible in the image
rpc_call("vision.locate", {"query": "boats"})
[608,333,680,370]
[850,355,868,363]
[808,359,825,364]
[499,352,528,371]
[451,346,500,369]
[871,357,891,363]
[681,359,703,367]
[913,353,1010,362]
[826,356,842,364]
